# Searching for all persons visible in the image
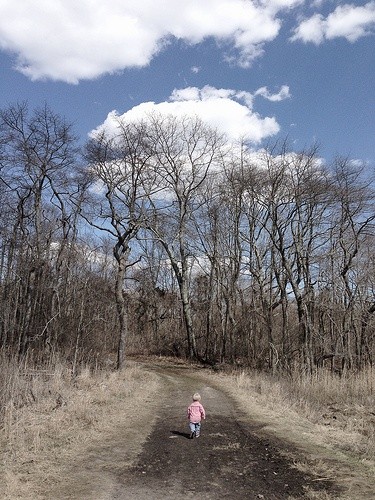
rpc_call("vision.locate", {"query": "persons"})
[189,390,206,440]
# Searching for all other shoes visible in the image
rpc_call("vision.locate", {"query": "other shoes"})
[190,431,194,439]
[195,434,200,438]
